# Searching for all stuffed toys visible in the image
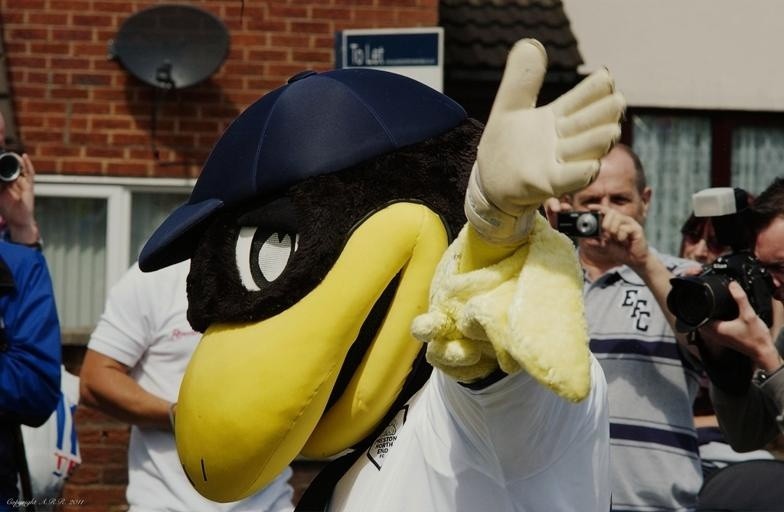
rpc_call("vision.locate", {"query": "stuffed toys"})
[140,37,627,512]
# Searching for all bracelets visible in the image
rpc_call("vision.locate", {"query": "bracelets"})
[16,237,45,251]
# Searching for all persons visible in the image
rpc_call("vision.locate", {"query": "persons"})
[79,260,298,512]
[679,185,756,479]
[0,118,79,512]
[695,176,784,509]
[541,144,709,510]
[0,241,63,510]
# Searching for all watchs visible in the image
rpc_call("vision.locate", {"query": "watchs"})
[758,363,783,382]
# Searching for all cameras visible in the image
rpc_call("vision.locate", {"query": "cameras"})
[667,254,758,335]
[0,151,24,184]
[558,210,601,238]
